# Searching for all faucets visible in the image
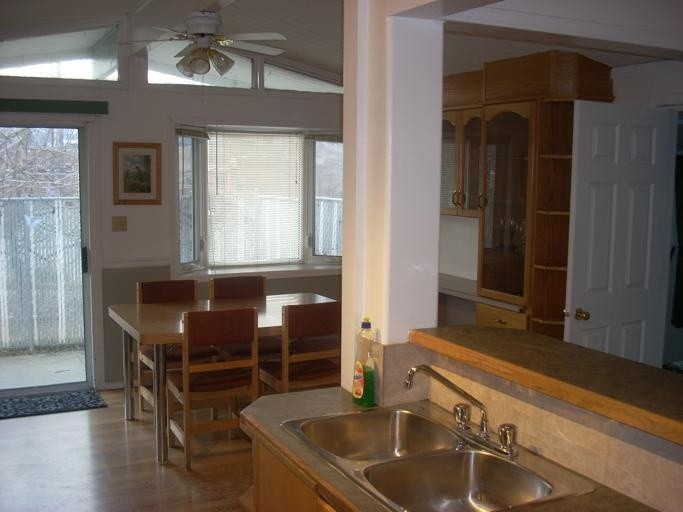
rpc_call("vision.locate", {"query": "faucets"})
[402,364,490,443]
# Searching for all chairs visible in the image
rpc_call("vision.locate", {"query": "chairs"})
[258,300,340,392]
[209,277,268,421]
[134,280,220,414]
[166,305,260,472]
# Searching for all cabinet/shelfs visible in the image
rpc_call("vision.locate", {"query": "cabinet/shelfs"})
[442,49,617,341]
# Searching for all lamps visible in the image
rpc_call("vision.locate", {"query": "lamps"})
[175,38,234,77]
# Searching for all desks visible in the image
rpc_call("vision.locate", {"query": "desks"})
[108,292,336,464]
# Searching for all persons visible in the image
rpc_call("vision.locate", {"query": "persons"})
[132,166,145,183]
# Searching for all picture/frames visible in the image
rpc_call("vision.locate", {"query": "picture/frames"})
[112,141,162,206]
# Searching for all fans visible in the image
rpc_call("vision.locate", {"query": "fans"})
[119,10,286,57]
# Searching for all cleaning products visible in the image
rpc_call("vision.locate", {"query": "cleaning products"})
[351,316,378,407]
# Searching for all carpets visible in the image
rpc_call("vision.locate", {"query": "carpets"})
[0,388,107,420]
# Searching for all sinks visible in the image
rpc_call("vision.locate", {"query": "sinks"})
[353,447,553,511]
[281,399,465,469]
